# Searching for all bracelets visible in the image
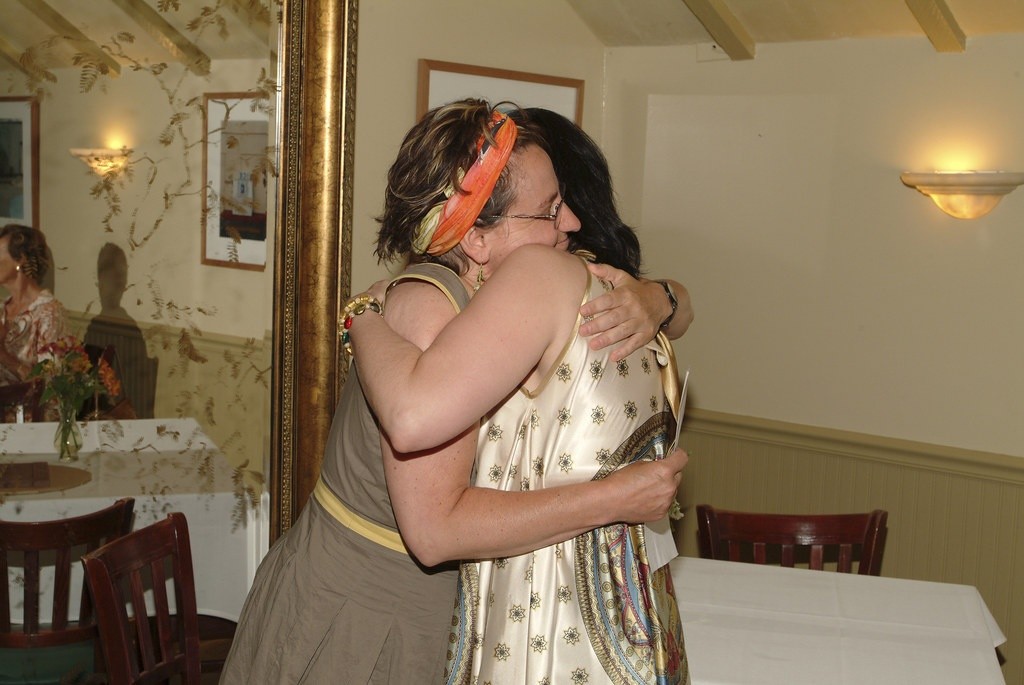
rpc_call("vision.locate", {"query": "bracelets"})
[336,295,384,354]
[16,362,24,378]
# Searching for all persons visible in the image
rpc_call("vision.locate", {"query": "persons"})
[218,96,693,684]
[0,223,70,386]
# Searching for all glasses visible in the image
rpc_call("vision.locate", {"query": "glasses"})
[480,183,566,229]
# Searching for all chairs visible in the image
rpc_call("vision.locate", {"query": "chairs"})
[697,501,888,578]
[79,512,205,684]
[0,497,135,684]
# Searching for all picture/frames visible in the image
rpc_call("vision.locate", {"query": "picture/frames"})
[0,96,41,230]
[202,90,272,271]
[415,54,585,127]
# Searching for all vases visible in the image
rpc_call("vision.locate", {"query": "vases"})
[53,397,83,462]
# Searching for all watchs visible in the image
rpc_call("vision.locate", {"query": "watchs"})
[646,280,678,328]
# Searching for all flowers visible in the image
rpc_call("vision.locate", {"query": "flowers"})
[24,336,120,411]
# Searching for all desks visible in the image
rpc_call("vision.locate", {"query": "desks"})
[0,462,91,499]
[0,415,260,622]
[668,554,1009,685]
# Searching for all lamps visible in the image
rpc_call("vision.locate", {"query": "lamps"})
[900,169,1024,218]
